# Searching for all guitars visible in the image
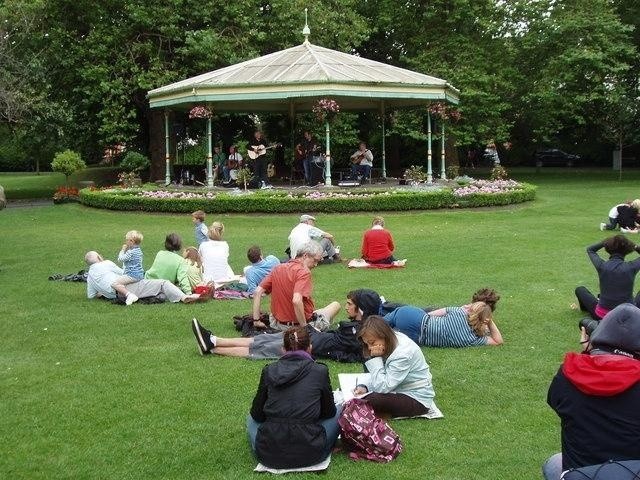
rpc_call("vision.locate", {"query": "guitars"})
[247,145,277,159]
[267,153,276,178]
[352,147,375,165]
[296,144,321,160]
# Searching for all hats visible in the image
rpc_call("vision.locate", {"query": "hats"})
[605,235,636,256]
[300,215,315,222]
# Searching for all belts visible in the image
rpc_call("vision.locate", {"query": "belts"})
[116,290,118,298]
[279,318,314,326]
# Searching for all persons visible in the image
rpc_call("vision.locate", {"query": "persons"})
[381,301,504,349]
[349,142,374,181]
[288,214,341,265]
[242,245,280,293]
[600,198,640,234]
[198,221,235,282]
[84,249,202,304]
[361,217,395,265]
[110,230,145,306]
[542,302,640,480]
[191,288,382,363]
[221,144,244,180]
[293,129,319,184]
[203,143,226,180]
[245,131,281,187]
[272,140,289,181]
[183,246,205,286]
[379,287,502,317]
[575,234,640,321]
[351,314,436,417]
[191,209,209,250]
[252,240,342,332]
[144,231,193,296]
[245,325,344,469]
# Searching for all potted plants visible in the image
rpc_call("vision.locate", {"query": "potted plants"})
[121,151,151,185]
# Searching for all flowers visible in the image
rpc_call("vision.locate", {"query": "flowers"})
[449,110,461,121]
[429,101,444,115]
[189,106,213,120]
[312,98,339,120]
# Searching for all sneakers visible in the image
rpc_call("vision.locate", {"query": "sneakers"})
[183,294,201,304]
[126,295,138,305]
[600,222,605,231]
[333,256,348,263]
[197,343,210,355]
[192,318,214,351]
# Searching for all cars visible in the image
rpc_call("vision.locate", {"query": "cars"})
[530,148,580,168]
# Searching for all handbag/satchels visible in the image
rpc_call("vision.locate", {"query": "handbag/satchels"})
[233,313,280,337]
[188,277,214,301]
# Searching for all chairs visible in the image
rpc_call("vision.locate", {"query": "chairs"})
[290,159,307,186]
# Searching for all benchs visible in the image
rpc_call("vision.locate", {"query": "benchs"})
[332,167,372,183]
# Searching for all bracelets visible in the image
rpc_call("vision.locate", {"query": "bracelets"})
[253,318,261,321]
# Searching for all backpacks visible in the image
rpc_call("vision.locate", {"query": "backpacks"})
[337,399,403,463]
